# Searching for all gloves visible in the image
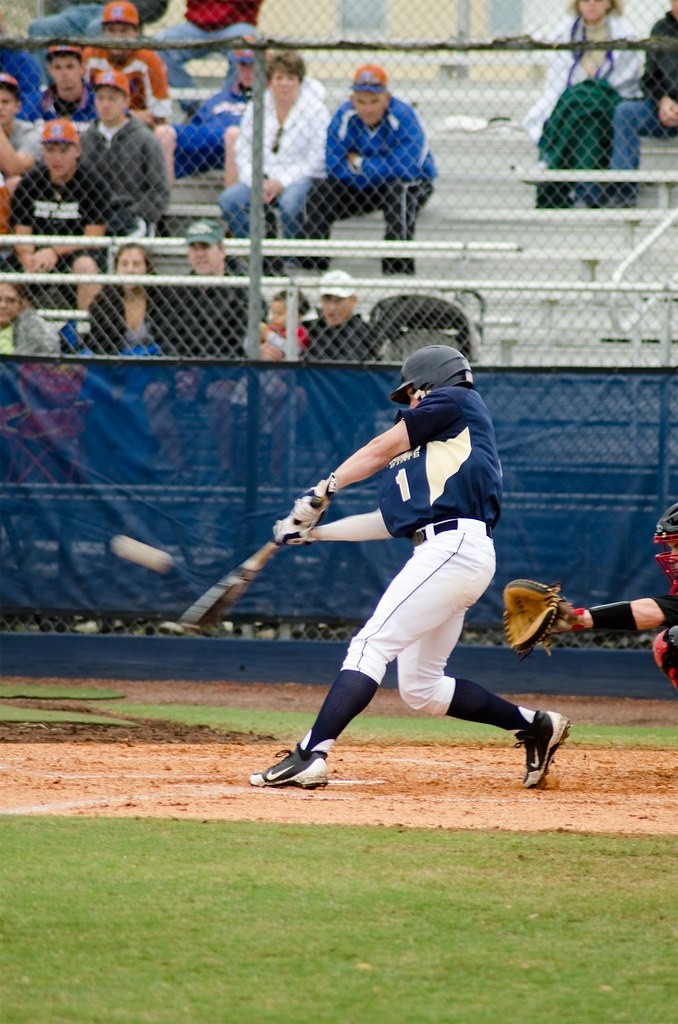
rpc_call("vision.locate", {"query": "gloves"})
[290,479,328,522]
[272,520,314,546]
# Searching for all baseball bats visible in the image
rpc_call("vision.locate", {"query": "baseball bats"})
[194,495,323,639]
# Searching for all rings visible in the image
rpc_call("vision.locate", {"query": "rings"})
[662,117,667,123]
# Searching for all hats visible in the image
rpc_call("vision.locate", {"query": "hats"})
[349,62,389,93]
[0,73,20,99]
[40,118,79,146]
[186,219,224,245]
[232,36,270,62]
[319,269,356,297]
[45,38,83,63]
[102,1,139,27]
[94,70,130,96]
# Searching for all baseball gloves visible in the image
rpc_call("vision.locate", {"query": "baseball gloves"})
[502,579,560,655]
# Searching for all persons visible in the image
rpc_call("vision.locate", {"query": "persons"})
[0,0,382,485]
[547,502,678,690]
[291,63,438,277]
[249,345,574,791]
[521,0,678,209]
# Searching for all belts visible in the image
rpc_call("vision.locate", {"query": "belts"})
[411,520,493,547]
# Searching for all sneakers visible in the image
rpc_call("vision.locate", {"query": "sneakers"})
[249,743,330,789]
[514,710,570,788]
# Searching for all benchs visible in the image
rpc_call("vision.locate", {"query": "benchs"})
[87,57,678,367]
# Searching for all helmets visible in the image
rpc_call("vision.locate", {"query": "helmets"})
[653,502,678,595]
[389,345,474,405]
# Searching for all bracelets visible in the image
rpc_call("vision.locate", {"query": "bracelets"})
[352,155,362,170]
[50,246,60,257]
